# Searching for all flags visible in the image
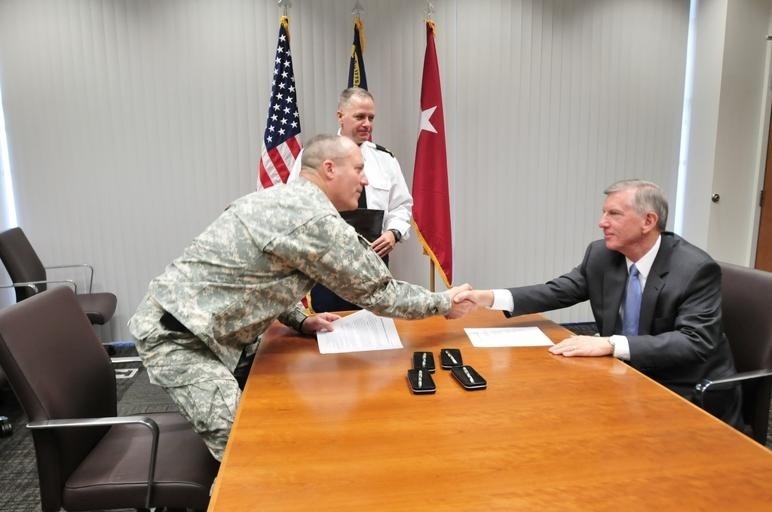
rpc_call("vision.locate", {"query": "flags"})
[411,20,454,289]
[257,15,311,309]
[347,18,368,90]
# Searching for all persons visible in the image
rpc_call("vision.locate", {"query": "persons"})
[127,138,474,465]
[451,179,734,403]
[286,85,414,308]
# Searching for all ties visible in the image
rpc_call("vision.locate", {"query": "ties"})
[359,185,367,208]
[620,264,643,336]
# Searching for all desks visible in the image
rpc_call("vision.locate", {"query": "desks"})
[203,301,771,511]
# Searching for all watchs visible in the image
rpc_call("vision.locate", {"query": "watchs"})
[388,229,401,242]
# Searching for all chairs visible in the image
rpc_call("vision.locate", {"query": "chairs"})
[685,258,772,448]
[0,285,218,512]
[0,228,117,325]
